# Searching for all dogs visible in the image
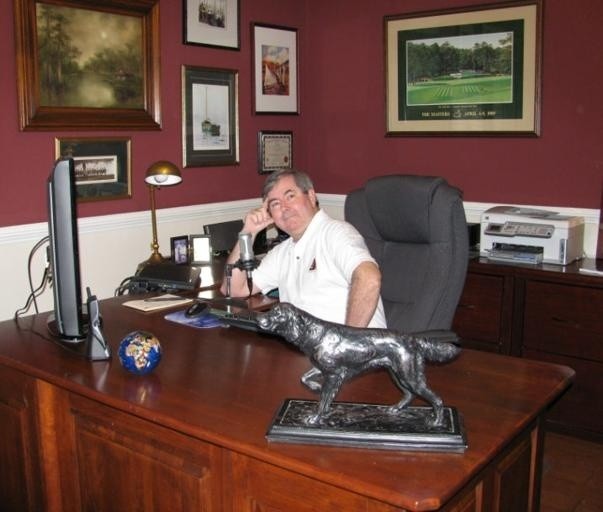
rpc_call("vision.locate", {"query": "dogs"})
[254,301,462,427]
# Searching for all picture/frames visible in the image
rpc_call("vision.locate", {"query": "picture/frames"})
[382,1,543,138]
[13,0,162,132]
[54,137,132,203]
[190,234,213,265]
[171,235,189,265]
[182,0,241,51]
[180,63,240,168]
[250,21,300,116]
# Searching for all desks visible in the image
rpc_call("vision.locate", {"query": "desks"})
[1,293,575,512]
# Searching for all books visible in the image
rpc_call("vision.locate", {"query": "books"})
[121,293,193,312]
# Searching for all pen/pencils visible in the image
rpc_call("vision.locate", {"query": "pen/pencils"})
[144,298,187,302]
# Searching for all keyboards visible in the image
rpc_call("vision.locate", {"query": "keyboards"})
[221,310,281,334]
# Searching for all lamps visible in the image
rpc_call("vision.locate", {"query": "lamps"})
[142,160,182,265]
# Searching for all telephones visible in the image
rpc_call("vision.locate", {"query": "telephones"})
[86,287,112,361]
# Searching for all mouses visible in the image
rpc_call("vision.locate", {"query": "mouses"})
[185,302,211,318]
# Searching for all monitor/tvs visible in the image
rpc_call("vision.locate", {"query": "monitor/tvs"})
[47,158,104,343]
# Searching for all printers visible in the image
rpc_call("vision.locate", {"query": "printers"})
[479,206,584,266]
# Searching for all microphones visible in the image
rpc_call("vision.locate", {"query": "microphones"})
[239,233,253,291]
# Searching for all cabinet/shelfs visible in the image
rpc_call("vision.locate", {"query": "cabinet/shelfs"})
[513,278,603,443]
[450,266,512,355]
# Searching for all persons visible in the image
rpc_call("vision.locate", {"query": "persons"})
[218,167,387,331]
